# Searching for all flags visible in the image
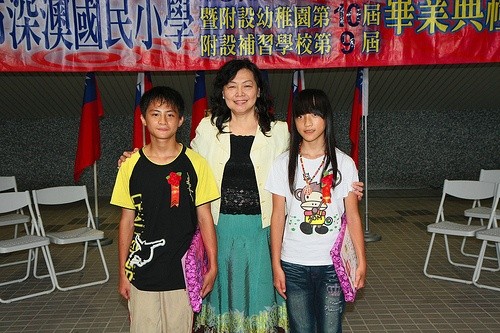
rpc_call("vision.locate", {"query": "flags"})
[287,68,306,132]
[133,71,154,152]
[349,66,368,169]
[190,70,208,142]
[258,69,274,115]
[73,71,104,182]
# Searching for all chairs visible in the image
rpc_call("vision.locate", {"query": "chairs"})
[423,169,500,291]
[0,175,110,304]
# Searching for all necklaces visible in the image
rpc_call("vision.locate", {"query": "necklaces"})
[298,141,327,194]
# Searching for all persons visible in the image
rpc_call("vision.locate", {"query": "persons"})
[109,86,221,333]
[117,57,364,333]
[264,88,367,333]
